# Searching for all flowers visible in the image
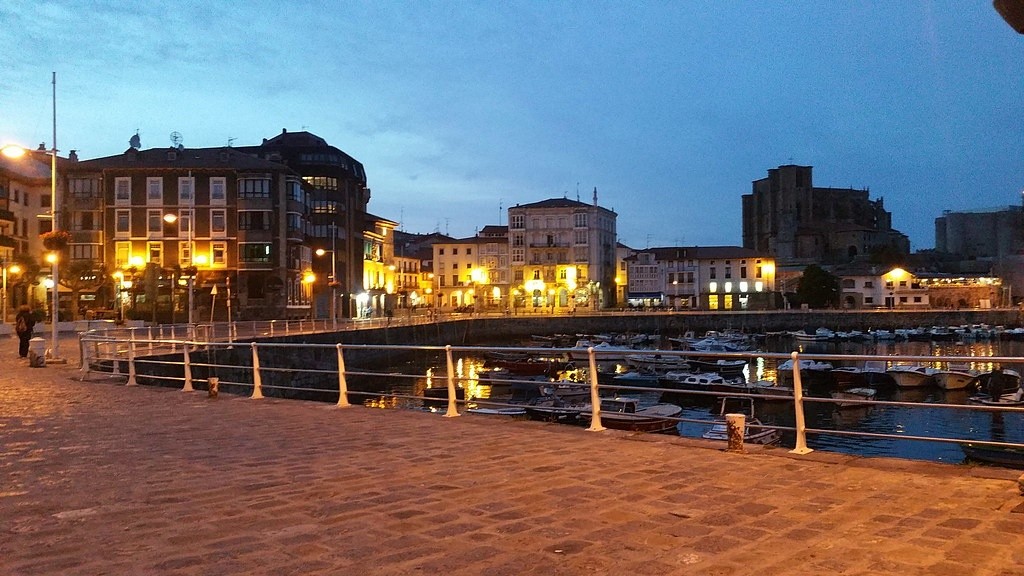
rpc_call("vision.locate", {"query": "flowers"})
[39,229,73,241]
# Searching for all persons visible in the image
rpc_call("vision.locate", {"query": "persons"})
[385,307,394,327]
[14,299,37,357]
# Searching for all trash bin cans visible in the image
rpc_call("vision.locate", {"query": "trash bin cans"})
[27,336,49,369]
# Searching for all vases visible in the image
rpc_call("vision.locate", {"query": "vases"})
[44,239,67,249]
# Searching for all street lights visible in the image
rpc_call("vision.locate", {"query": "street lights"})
[550,290,554,314]
[427,288,430,309]
[3,265,19,322]
[429,274,440,292]
[3,144,66,364]
[317,249,337,329]
[469,290,472,316]
[114,273,123,321]
[163,214,193,323]
[514,290,518,315]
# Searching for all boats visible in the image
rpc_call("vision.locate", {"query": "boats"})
[468,386,591,422]
[420,322,1024,469]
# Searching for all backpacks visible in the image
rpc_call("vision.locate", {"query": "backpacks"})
[16,316,27,334]
